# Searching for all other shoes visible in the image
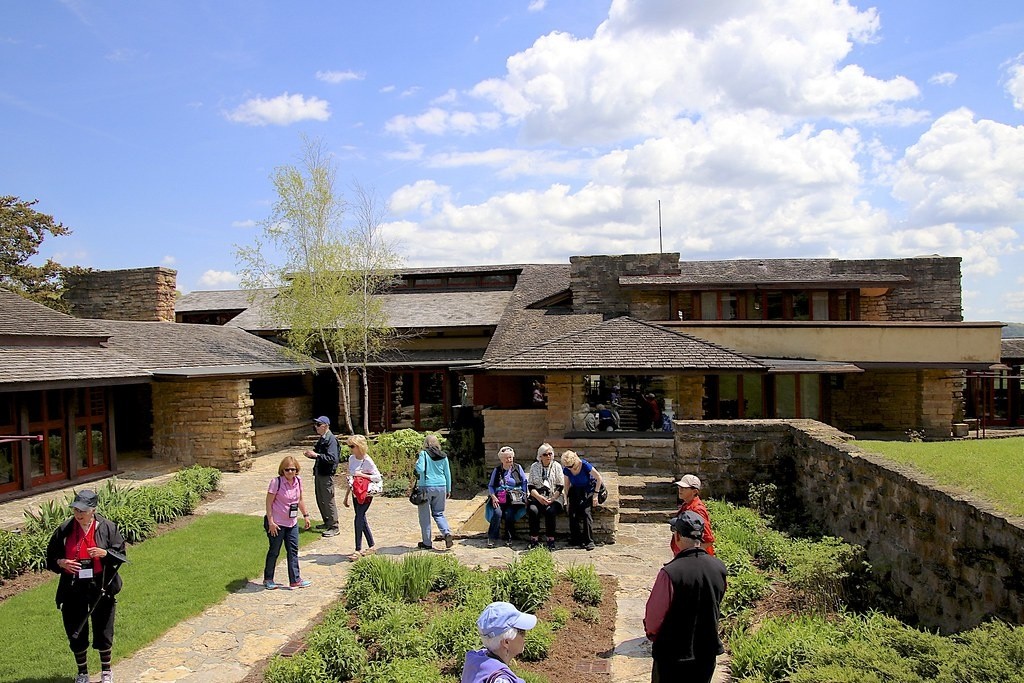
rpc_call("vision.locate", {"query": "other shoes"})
[346,554,358,561]
[360,549,376,555]
[418,542,432,549]
[445,533,453,548]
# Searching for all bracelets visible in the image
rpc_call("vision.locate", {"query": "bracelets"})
[303,513,309,518]
[594,490,599,493]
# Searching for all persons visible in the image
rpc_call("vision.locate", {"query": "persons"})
[487,447,527,548]
[415,434,453,550]
[577,385,659,432]
[670,474,715,558]
[47,490,126,683]
[343,435,381,560]
[263,456,311,590]
[527,443,564,550]
[303,416,341,537]
[643,511,728,683]
[461,602,537,683]
[562,450,602,550]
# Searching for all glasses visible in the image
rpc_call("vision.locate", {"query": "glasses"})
[284,468,297,472]
[314,423,322,427]
[542,453,552,456]
[348,445,357,449]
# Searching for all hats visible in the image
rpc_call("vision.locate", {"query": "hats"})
[68,490,98,511]
[477,602,537,639]
[669,510,704,539]
[675,474,701,490]
[314,416,330,425]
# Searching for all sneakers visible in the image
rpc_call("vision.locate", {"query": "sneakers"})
[316,524,330,529]
[263,579,278,590]
[76,674,89,683]
[289,580,312,589]
[322,528,340,537]
[488,536,595,551]
[101,670,112,683]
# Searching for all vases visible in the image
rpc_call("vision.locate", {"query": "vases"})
[661,398,676,432]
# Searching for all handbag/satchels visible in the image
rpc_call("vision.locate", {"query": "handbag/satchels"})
[598,487,608,504]
[410,488,428,505]
[264,515,271,538]
